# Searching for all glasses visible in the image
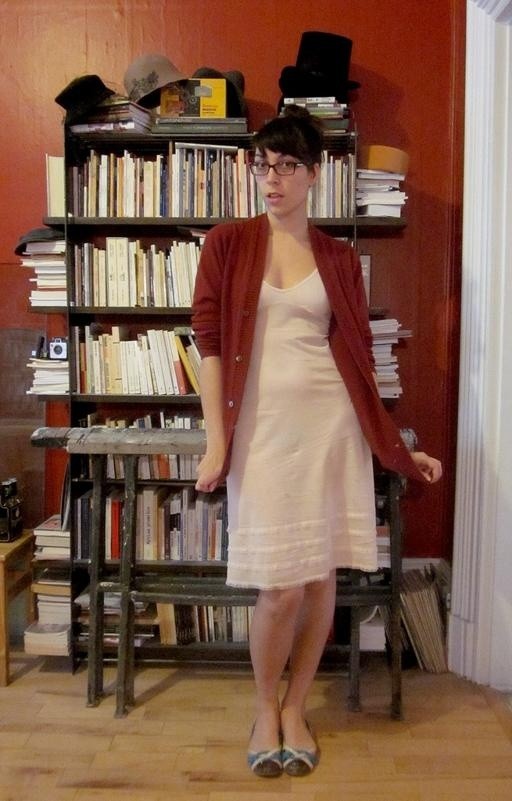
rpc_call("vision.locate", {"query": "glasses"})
[249,162,305,176]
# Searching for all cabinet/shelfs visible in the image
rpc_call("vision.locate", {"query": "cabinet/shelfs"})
[28,97,407,675]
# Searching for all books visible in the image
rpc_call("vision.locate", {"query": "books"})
[360,253,372,309]
[72,76,350,139]
[308,145,410,218]
[20,240,71,656]
[338,319,450,675]
[72,142,267,661]
[45,154,65,216]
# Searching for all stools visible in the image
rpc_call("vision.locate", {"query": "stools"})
[0,530,33,687]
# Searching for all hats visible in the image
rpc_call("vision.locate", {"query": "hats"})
[282,32,360,88]
[14,227,65,256]
[125,55,188,109]
[55,75,115,123]
[192,68,248,118]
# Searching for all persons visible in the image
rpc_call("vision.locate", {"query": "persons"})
[188,101,445,776]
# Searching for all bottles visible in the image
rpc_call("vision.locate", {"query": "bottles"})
[0,478,24,544]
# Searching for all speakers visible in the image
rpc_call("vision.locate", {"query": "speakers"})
[48,337,68,360]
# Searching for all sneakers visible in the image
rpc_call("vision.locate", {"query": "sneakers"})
[247,719,283,777]
[282,719,317,776]
